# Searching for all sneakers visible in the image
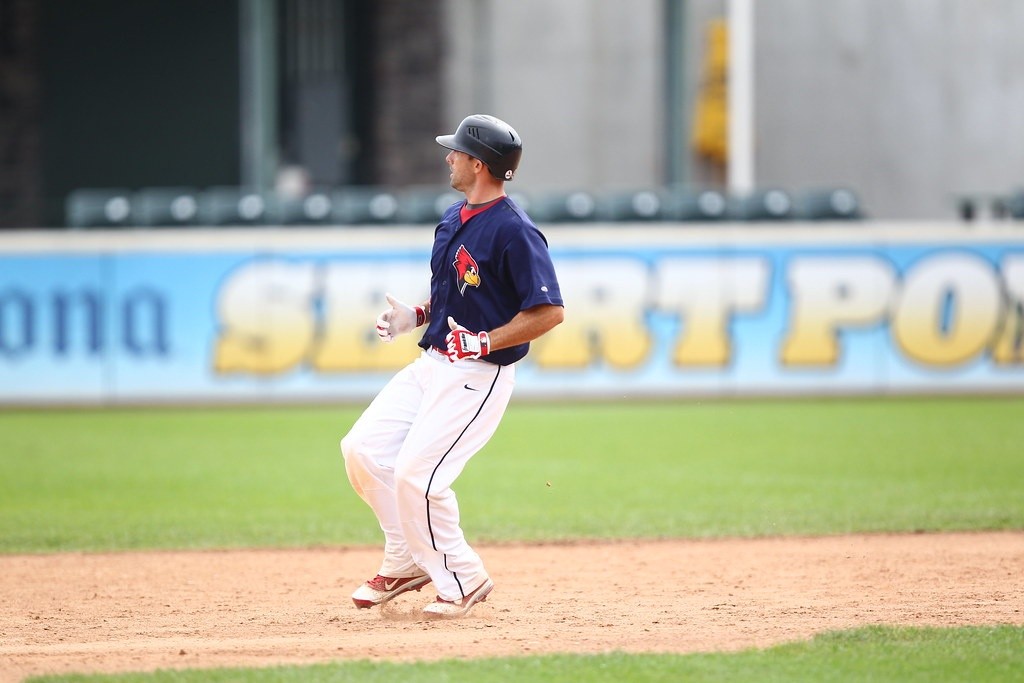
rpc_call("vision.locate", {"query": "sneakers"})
[423,576,495,619]
[352,574,432,609]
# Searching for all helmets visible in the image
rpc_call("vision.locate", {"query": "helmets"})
[435,114,523,180]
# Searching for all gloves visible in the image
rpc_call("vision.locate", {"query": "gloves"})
[444,316,491,364]
[376,293,426,343]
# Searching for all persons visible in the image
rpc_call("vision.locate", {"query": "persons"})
[338,115,566,614]
